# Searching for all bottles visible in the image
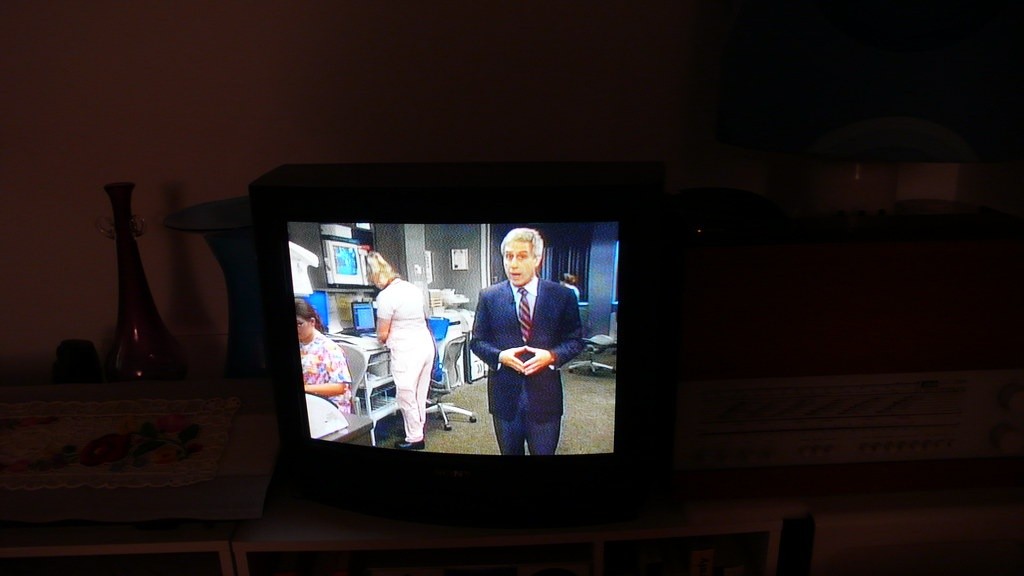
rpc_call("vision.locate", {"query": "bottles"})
[96,182,181,379]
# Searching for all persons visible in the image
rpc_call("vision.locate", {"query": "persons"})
[367,250,436,449]
[295,297,352,414]
[469,227,584,455]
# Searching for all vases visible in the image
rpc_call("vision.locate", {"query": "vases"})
[96,182,182,382]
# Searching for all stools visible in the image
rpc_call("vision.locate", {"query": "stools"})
[567,334,617,377]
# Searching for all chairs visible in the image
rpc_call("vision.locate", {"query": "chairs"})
[334,343,368,417]
[426,333,478,432]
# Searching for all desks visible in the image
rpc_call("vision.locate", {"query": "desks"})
[0,377,782,576]
[315,413,374,446]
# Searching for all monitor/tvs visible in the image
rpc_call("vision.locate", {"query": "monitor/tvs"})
[247,158,680,527]
